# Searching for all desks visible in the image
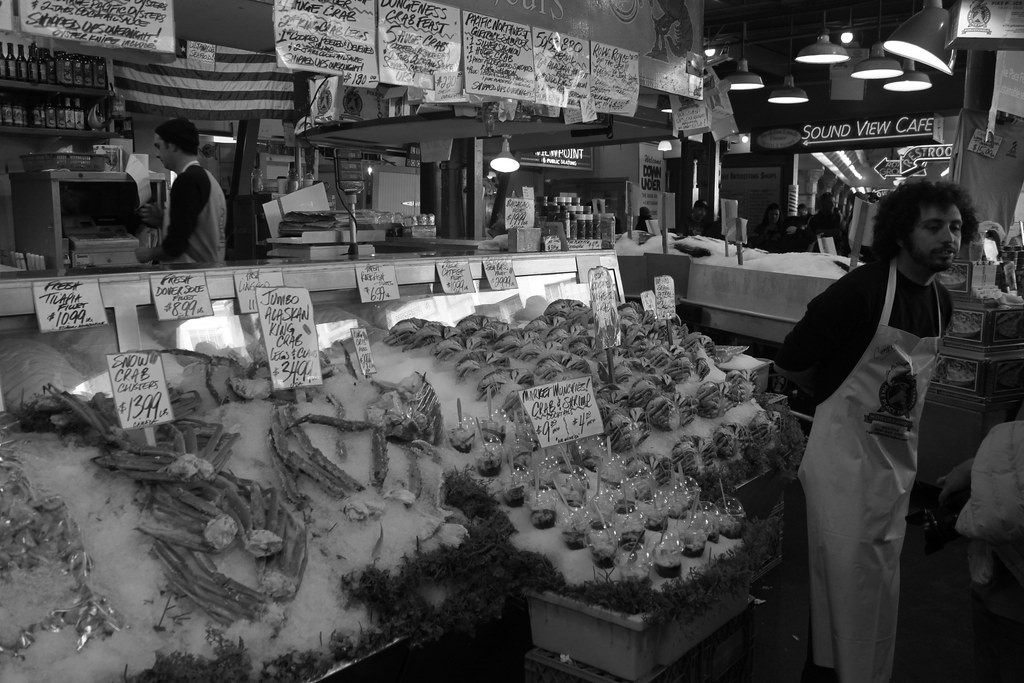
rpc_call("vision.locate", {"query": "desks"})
[9,172,166,272]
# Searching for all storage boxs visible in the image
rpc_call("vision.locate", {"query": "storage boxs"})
[916,260,1024,488]
[524,546,753,683]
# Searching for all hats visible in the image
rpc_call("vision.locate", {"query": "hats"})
[694,200,708,209]
[640,207,650,215]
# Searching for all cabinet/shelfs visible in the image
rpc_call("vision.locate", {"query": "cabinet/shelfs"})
[0,55,120,138]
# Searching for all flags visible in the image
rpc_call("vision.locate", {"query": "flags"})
[114,38,296,120]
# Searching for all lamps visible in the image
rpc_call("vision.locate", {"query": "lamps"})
[489,137,520,173]
[724,0,956,104]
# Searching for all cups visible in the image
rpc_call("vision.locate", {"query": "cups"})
[446,403,746,591]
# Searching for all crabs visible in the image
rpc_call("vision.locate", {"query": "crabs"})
[16,347,441,633]
[381,300,778,486]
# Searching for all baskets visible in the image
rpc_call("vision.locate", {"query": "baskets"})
[20,152,107,172]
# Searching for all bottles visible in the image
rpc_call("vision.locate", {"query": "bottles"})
[57,94,85,130]
[304,172,313,188]
[601,213,615,249]
[541,197,601,240]
[55,51,106,88]
[0,89,56,129]
[0,41,54,84]
[251,166,264,192]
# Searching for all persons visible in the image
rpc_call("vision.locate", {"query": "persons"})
[633,206,654,232]
[774,177,979,683]
[742,192,851,259]
[680,199,720,239]
[134,117,227,270]
